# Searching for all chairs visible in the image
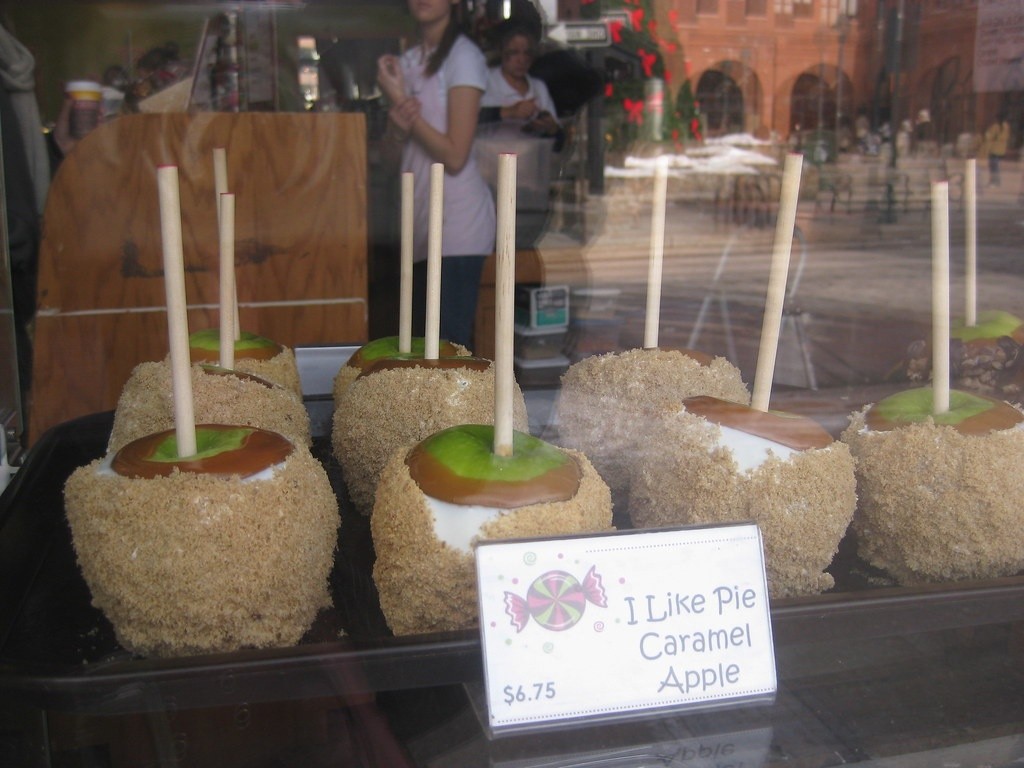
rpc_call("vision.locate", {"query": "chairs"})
[686,224,824,392]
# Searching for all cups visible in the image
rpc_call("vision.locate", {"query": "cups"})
[63,81,103,139]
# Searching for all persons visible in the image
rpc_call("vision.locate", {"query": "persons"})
[374,0,498,354]
[983,112,1009,188]
[474,20,566,206]
[54,10,274,158]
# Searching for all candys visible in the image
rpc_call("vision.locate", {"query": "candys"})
[62,307,1024,662]
